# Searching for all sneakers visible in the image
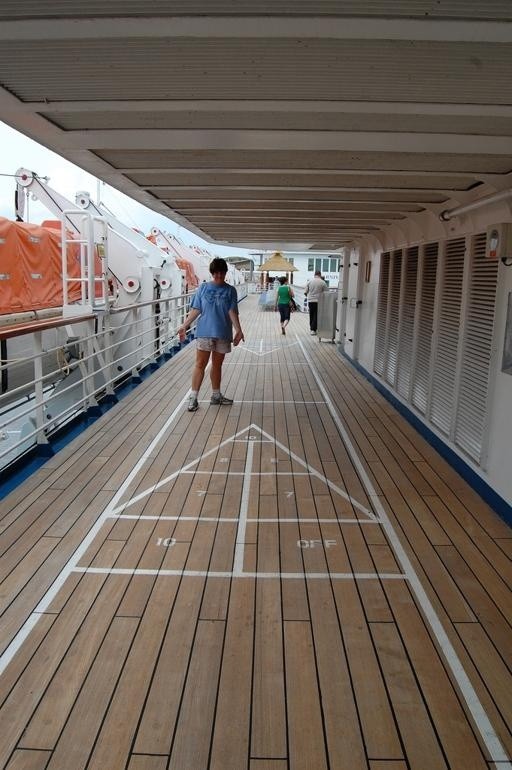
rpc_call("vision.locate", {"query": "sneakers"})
[210,392,234,405]
[310,330,316,335]
[187,396,199,412]
[281,327,285,335]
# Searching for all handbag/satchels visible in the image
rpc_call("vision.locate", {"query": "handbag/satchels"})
[288,296,297,313]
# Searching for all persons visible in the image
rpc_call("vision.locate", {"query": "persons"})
[273,276,294,336]
[175,258,246,411]
[304,271,327,335]
